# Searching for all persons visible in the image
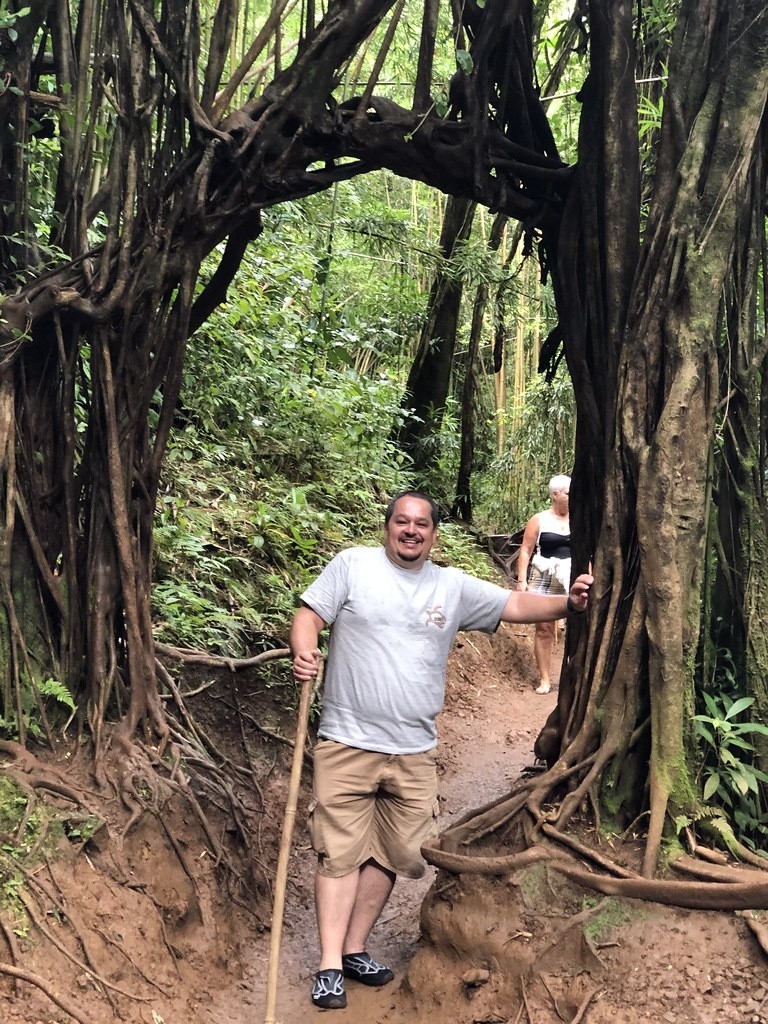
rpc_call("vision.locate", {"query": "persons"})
[290,491,594,1009]
[516,475,572,694]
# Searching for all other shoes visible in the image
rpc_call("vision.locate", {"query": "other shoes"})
[311,968,346,1009]
[342,952,394,986]
[536,683,551,695]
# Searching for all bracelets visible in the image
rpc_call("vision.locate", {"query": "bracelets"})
[567,597,585,616]
